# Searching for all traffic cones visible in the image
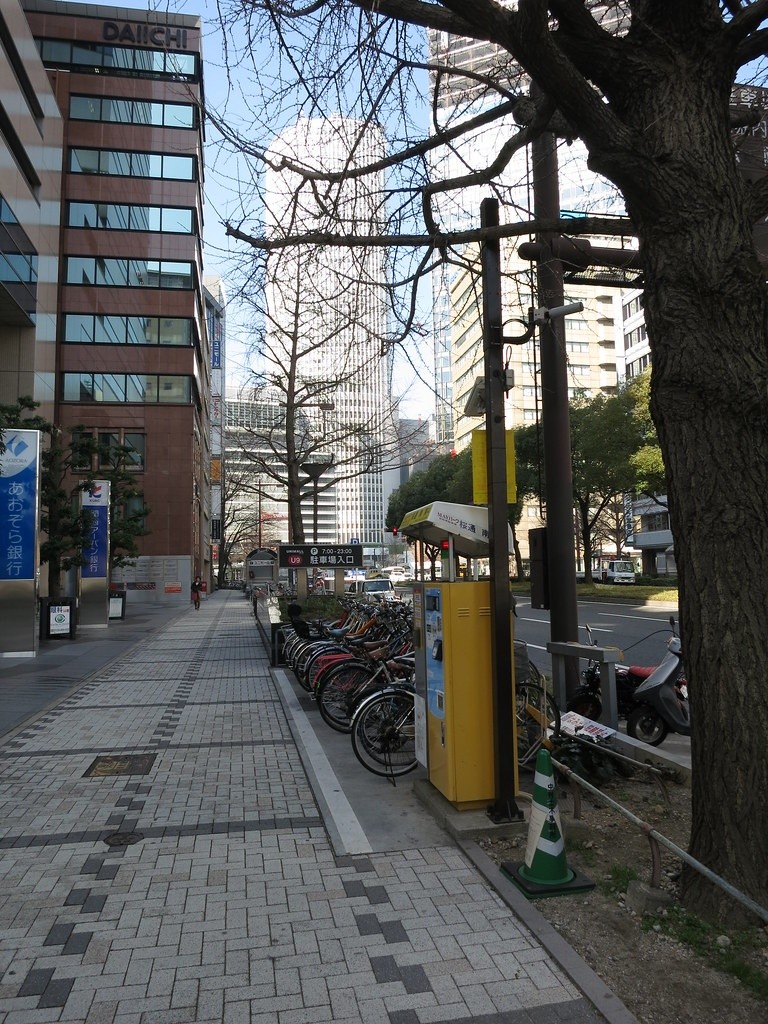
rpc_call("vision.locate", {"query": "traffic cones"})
[498,748,598,901]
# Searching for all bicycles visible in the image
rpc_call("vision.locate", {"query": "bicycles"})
[279,583,563,790]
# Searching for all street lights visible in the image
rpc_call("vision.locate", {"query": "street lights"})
[301,463,330,543]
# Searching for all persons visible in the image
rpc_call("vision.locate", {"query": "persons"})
[190,576,201,609]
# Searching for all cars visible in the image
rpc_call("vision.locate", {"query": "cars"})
[319,561,443,582]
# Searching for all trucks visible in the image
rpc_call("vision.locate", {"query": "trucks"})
[576,560,636,584]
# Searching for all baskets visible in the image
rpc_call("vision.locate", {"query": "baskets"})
[513,638,529,683]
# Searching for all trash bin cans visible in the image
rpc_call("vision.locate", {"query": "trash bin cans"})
[109,592,126,621]
[38,596,76,642]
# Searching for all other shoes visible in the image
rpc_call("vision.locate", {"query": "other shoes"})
[195,607,199,609]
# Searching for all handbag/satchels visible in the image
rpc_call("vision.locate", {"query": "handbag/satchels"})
[191,591,197,600]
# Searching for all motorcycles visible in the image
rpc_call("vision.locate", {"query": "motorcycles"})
[564,616,691,749]
